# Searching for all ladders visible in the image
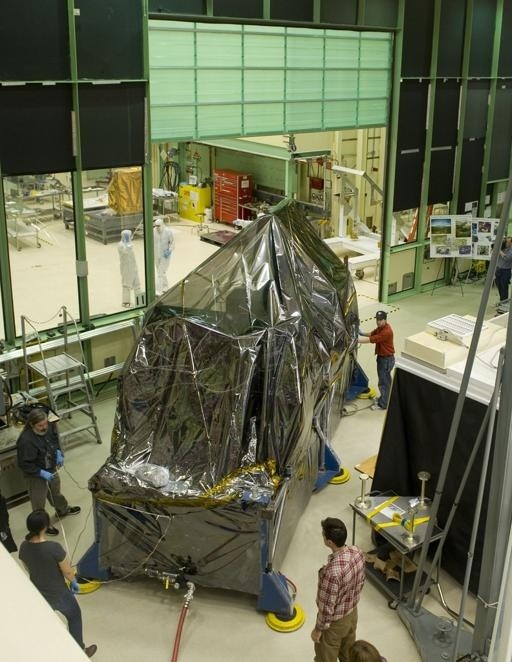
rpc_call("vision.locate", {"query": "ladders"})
[21,306,102,451]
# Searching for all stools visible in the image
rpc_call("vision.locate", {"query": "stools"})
[195,213,206,231]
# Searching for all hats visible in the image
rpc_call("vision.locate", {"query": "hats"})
[374,310,387,319]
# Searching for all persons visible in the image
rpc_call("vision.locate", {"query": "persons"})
[18,409,81,536]
[492,235,512,301]
[118,228,142,308]
[353,310,396,411]
[17,509,99,658]
[152,218,175,298]
[309,516,359,662]
[349,639,387,662]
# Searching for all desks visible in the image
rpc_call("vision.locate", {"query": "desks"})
[240,205,257,220]
[349,494,444,608]
[152,189,178,215]
[5,180,143,251]
[0,415,29,505]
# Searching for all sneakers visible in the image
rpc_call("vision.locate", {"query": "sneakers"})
[55,505,82,520]
[370,403,384,410]
[84,644,98,658]
[45,525,59,537]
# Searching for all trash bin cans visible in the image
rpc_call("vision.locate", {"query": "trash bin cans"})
[204,207,213,223]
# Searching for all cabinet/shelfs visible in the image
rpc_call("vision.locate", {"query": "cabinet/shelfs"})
[213,168,253,226]
[179,183,211,222]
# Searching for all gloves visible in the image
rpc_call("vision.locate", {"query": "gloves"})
[55,449,64,468]
[358,328,364,336]
[39,469,56,481]
[69,579,80,593]
